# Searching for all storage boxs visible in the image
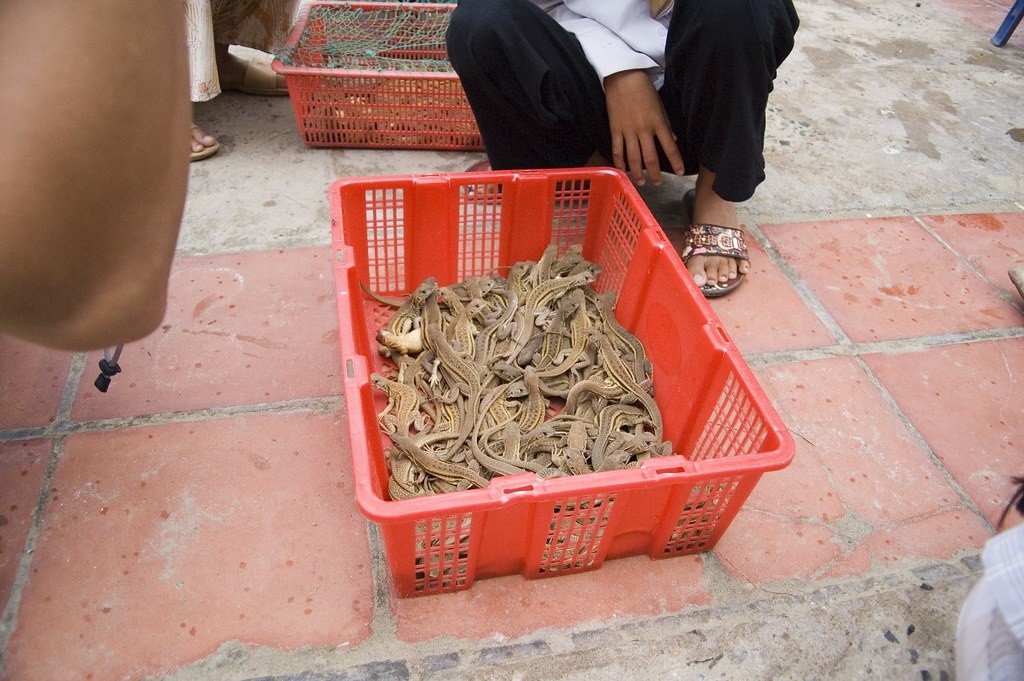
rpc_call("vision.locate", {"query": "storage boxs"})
[270,2,490,152]
[328,164,796,602]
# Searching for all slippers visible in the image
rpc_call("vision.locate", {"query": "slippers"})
[681,187,751,297]
[188,125,220,161]
[458,150,613,205]
[215,59,290,97]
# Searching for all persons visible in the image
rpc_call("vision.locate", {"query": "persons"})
[445,0,800,298]
[184,0,301,153]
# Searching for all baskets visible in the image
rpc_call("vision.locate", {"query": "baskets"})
[271,0,487,151]
[331,164,794,598]
